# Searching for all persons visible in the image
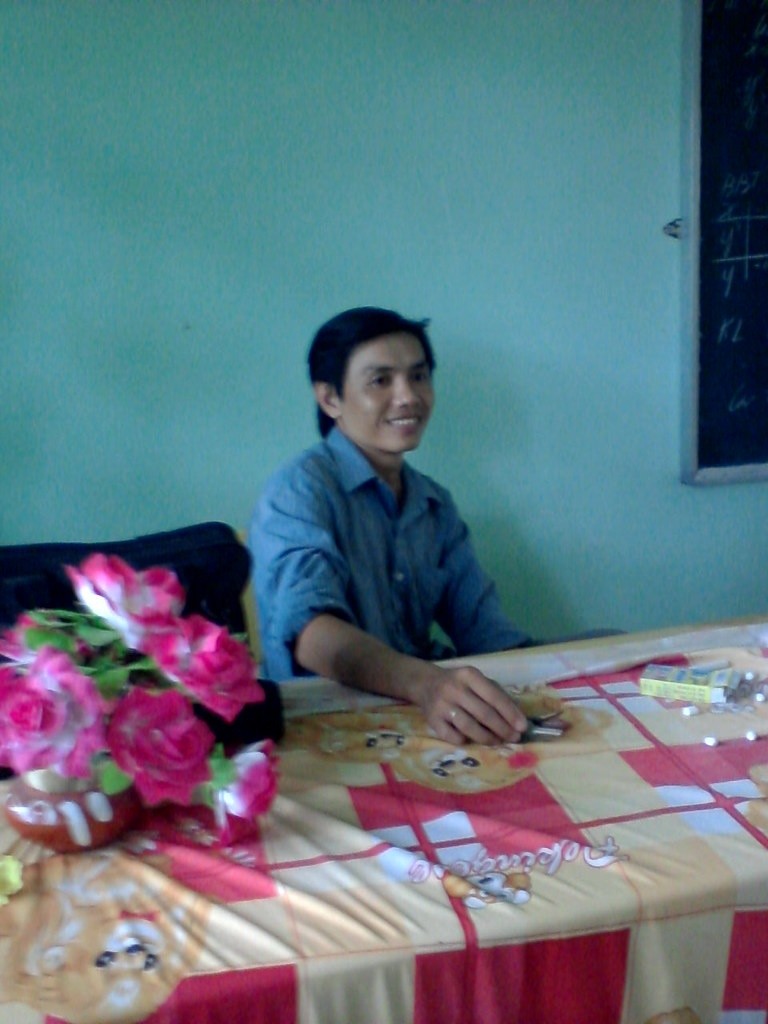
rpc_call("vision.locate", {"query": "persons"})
[246,306,627,746]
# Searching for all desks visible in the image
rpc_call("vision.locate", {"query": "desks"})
[0,614,768,1024]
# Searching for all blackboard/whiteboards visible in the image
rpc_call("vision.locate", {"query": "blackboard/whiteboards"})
[680,0,768,484]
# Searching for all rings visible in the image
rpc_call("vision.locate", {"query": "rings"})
[450,710,459,719]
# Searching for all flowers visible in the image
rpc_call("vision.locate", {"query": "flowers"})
[0,553,280,845]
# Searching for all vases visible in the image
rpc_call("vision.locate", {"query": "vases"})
[4,765,143,854]
